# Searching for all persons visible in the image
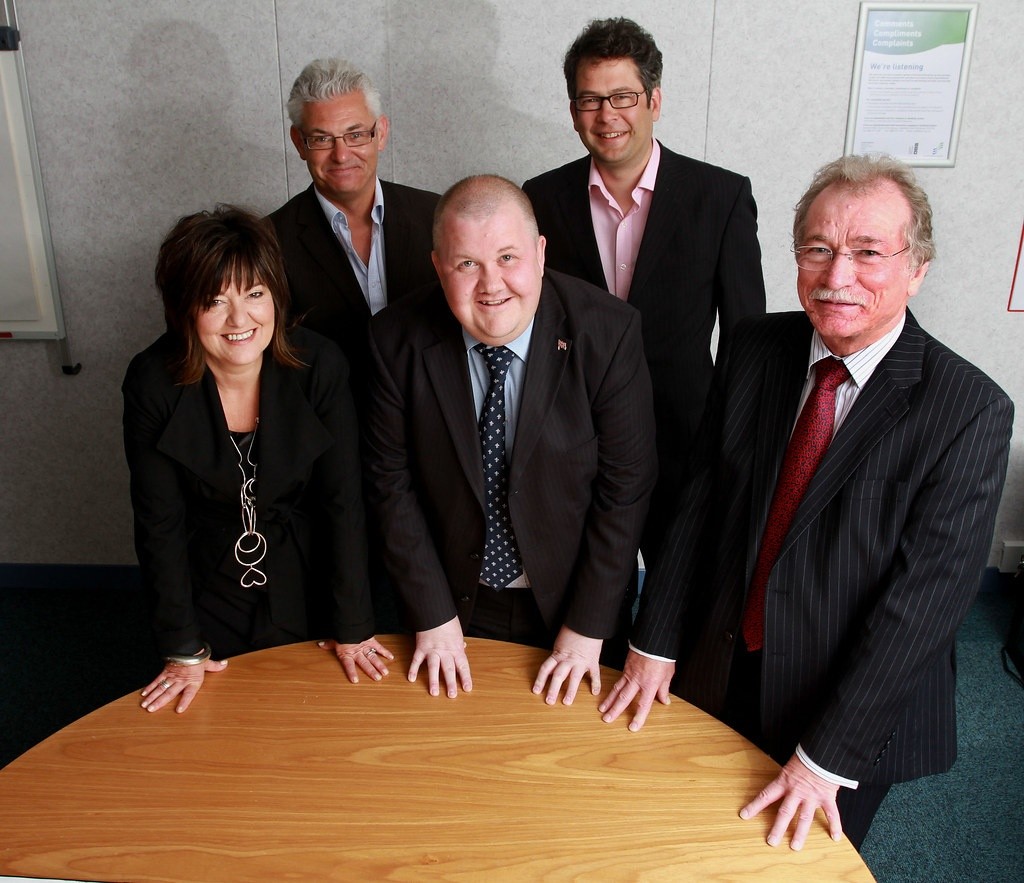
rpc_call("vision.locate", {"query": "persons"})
[255,58,449,632]
[121,202,396,713]
[524,15,766,574]
[598,155,1015,851]
[361,173,653,706]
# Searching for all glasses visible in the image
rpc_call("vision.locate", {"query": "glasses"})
[570,88,647,112]
[298,120,378,150]
[789,240,911,274]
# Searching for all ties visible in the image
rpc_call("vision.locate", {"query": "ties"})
[741,357,851,653]
[471,342,524,594]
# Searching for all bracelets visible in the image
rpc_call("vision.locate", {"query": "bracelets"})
[163,643,212,667]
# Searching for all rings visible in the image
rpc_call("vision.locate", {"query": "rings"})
[366,649,376,657]
[162,681,170,689]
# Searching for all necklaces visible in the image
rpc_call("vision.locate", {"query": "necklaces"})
[229,417,269,588]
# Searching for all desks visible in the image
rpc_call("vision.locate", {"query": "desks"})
[0,632,878,883]
